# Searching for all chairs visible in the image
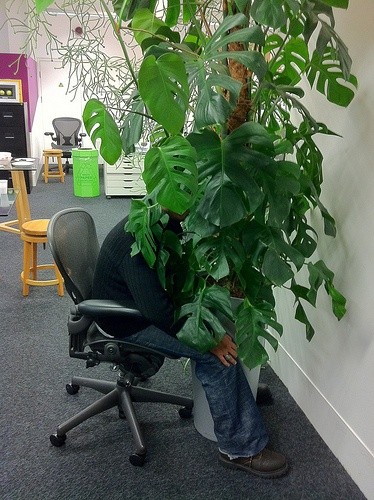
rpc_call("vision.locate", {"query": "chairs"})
[44,117,86,175]
[47,207,195,468]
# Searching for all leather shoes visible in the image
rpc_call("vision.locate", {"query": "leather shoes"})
[250,381,275,405]
[217,448,291,479]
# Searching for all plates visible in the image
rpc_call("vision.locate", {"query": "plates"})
[12,162,34,168]
[14,158,36,162]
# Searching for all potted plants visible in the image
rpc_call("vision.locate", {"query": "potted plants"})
[35,1,358,442]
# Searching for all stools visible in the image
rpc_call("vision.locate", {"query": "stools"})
[42,150,66,184]
[20,219,64,297]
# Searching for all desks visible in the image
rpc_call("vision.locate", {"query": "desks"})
[0,158,39,232]
[0,189,20,216]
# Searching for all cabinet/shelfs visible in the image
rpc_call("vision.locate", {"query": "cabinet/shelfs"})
[0,102,33,194]
[104,152,147,198]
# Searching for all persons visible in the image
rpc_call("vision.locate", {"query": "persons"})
[92,170,292,478]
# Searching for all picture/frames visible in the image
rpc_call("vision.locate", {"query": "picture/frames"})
[0,79,23,104]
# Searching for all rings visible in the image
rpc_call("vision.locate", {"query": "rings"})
[225,355,229,360]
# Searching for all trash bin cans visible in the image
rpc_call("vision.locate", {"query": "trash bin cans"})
[71,147,100,198]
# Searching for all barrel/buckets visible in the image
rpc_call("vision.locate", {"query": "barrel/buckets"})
[72,147,100,198]
